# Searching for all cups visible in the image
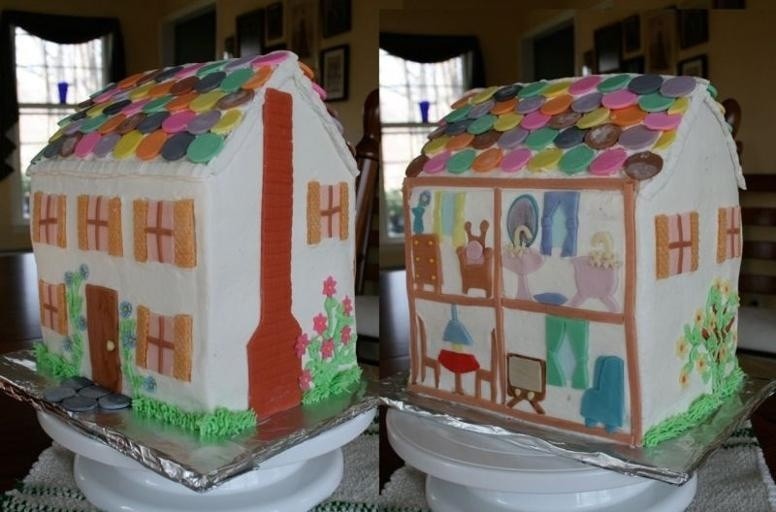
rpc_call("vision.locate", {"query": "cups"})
[58,81,69,104]
[419,100,429,121]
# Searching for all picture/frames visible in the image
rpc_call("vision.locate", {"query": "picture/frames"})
[318,42,350,102]
[709,0,747,12]
[234,0,287,57]
[593,4,708,79]
[320,0,351,38]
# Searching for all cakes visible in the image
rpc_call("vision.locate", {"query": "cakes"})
[400,71,748,447]
[27,46,362,436]
